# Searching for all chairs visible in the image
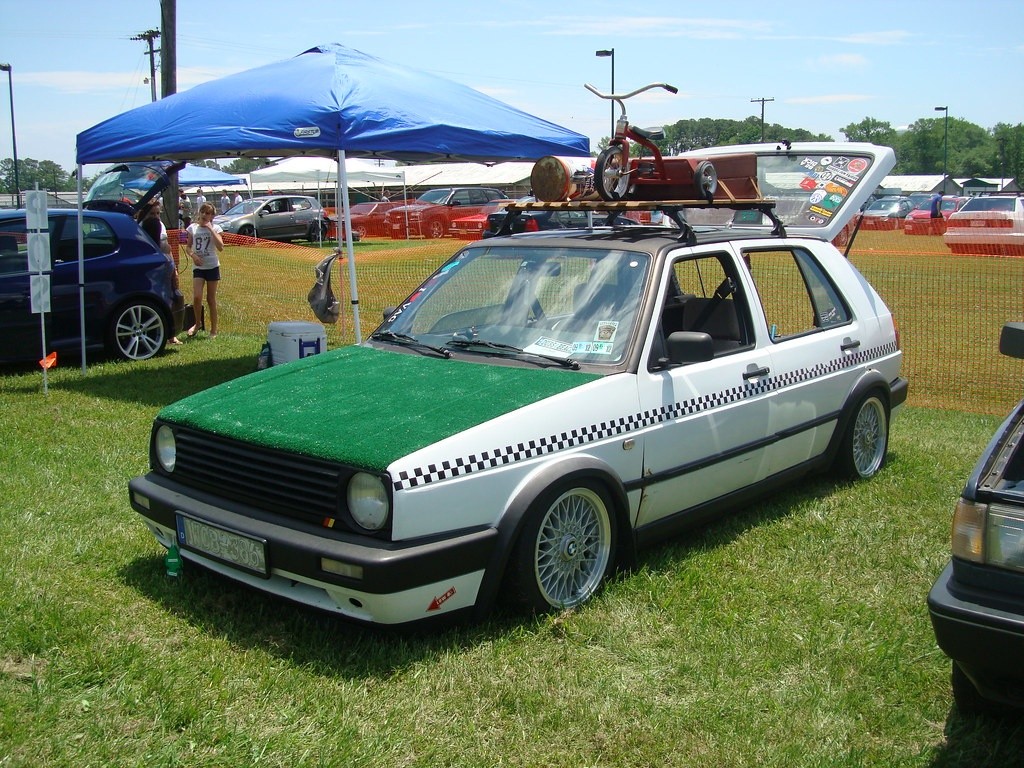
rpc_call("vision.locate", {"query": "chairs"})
[0,235,20,275]
[681,298,744,354]
[544,283,615,337]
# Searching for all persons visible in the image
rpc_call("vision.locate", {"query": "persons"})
[76,45,590,373]
[159,188,207,229]
[381,190,391,202]
[268,187,284,211]
[221,188,243,214]
[931,191,945,218]
[139,202,183,345]
[186,201,223,339]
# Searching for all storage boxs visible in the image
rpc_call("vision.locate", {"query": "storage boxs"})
[266,321,327,366]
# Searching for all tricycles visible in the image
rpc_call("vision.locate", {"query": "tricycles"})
[584,83,717,202]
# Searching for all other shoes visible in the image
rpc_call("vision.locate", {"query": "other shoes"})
[167,336,183,344]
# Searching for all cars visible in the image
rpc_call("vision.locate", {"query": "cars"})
[128,141,910,638]
[926,321,1024,719]
[855,193,1024,255]
[449,199,516,240]
[482,195,643,239]
[763,197,855,246]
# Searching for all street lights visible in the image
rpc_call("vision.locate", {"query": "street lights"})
[0,63,20,209]
[935,106,948,192]
[596,48,614,139]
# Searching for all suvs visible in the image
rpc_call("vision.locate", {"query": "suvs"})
[383,187,509,239]
[0,209,186,361]
[342,202,405,239]
[212,195,330,242]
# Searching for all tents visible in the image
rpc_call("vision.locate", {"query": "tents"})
[120,164,252,246]
[250,154,409,245]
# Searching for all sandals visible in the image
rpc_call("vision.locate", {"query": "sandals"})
[205,332,218,341]
[188,321,202,336]
